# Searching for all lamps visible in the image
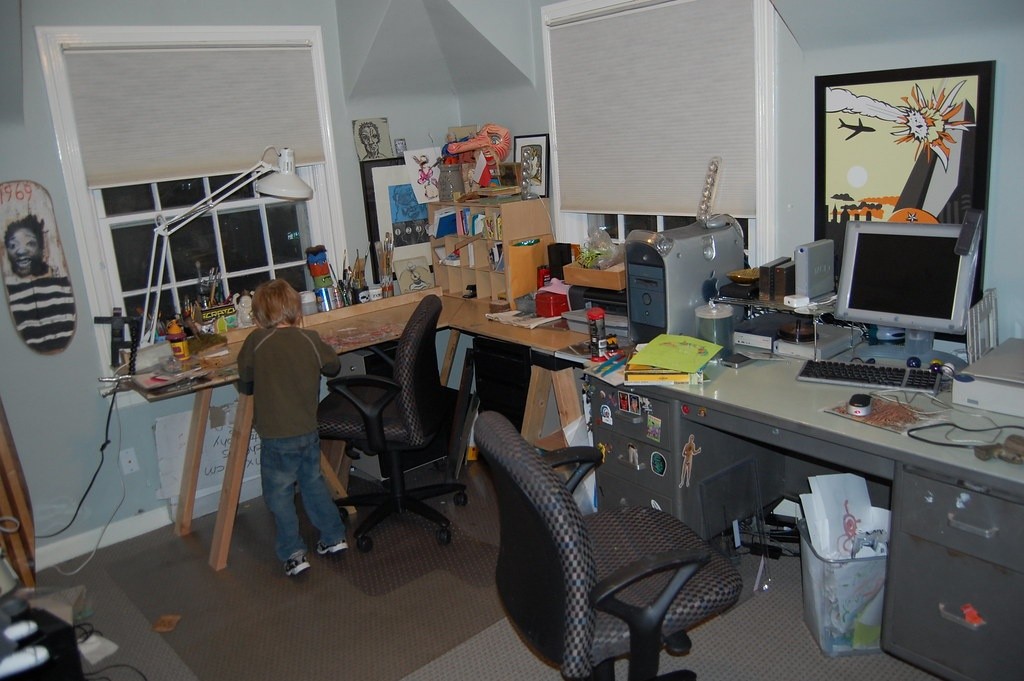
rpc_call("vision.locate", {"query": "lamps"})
[137,145,315,366]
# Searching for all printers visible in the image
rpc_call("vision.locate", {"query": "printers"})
[562,285,629,339]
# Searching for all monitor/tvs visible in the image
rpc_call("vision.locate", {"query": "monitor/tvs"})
[832,220,979,368]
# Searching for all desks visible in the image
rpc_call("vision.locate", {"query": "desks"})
[122,282,1024,681]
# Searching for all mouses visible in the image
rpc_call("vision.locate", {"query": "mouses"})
[848,393,874,416]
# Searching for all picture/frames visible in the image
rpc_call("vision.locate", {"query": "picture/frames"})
[815,56,997,346]
[361,157,440,284]
[512,133,549,198]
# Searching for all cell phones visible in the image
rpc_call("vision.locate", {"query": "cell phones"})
[721,353,756,368]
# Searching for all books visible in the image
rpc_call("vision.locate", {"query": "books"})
[624,343,711,386]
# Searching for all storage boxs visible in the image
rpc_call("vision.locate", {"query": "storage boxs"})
[562,251,627,290]
[952,338,1024,419]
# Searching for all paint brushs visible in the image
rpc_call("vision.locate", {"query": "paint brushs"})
[330,241,371,307]
[182,294,197,323]
[374,232,395,285]
[198,264,234,309]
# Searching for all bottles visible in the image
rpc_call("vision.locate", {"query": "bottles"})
[537,265,551,289]
[299,290,317,316]
[166,319,191,361]
[588,307,607,362]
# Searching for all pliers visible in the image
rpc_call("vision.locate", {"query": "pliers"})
[594,346,636,377]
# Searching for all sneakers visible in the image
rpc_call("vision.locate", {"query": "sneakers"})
[317,537,349,556]
[284,554,311,576]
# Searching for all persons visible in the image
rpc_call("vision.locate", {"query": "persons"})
[236,278,350,577]
[432,207,503,273]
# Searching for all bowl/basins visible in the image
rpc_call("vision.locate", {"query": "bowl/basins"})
[726,268,760,286]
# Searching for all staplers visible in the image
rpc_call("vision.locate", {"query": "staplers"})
[463,284,477,299]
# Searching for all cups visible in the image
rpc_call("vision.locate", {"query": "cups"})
[349,286,370,305]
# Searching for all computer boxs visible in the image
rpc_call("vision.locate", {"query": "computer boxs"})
[624,213,745,349]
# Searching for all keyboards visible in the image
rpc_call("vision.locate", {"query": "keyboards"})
[795,357,941,395]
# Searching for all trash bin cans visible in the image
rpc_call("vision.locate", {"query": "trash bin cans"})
[796,516,888,656]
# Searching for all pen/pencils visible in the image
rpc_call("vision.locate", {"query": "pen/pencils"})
[795,319,802,343]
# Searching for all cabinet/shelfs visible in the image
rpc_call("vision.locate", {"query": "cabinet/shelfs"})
[429,199,549,308]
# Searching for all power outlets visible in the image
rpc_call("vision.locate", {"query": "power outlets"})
[118,447,142,477]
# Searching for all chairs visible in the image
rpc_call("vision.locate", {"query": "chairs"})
[478,412,744,679]
[300,294,478,557]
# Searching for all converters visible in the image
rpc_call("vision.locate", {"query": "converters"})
[768,529,800,544]
[750,543,783,561]
